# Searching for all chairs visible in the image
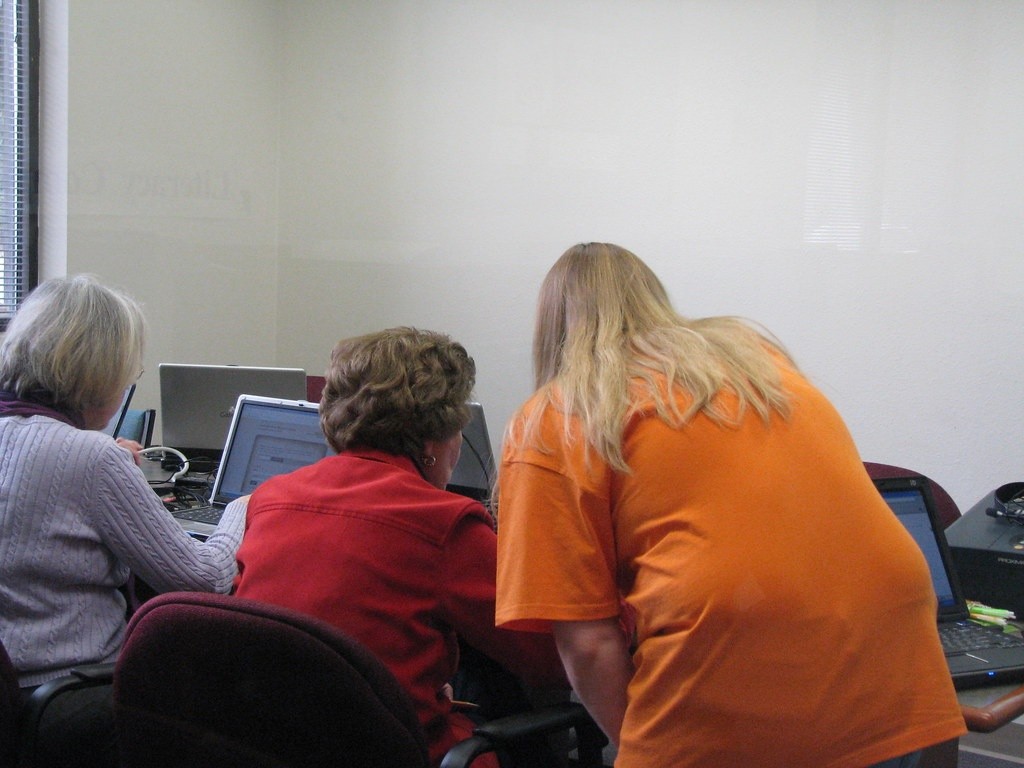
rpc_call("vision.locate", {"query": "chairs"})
[0,589,605,768]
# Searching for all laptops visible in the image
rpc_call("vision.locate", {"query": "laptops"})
[169,394,337,542]
[158,364,306,472]
[870,474,1024,692]
[445,403,498,507]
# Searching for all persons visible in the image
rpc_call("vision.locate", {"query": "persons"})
[224,325,574,768]
[0,272,250,705]
[497,241,969,767]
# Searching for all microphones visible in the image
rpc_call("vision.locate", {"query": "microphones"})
[985,508,1019,519]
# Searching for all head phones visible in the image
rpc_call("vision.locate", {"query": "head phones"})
[994,482,1024,526]
